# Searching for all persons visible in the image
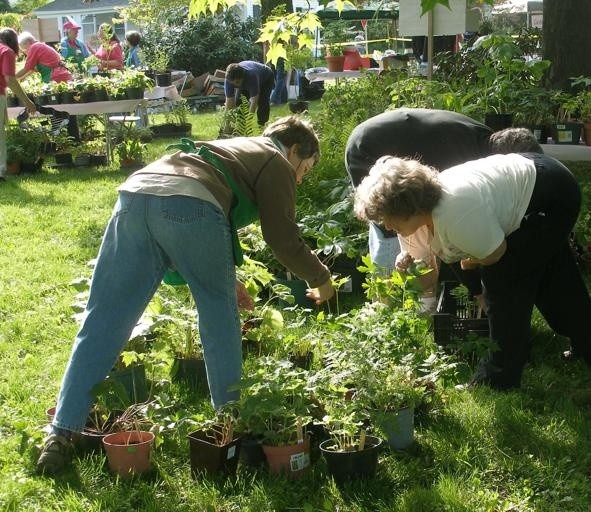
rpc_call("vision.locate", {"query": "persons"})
[352,153,590,393]
[0,27,37,182]
[15,31,83,148]
[58,22,91,74]
[343,107,547,297]
[35,113,337,478]
[86,23,124,146]
[221,61,276,128]
[124,31,149,128]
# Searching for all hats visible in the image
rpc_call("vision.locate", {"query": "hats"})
[64,21,81,30]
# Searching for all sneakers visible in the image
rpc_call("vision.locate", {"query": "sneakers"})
[454,368,520,394]
[562,335,590,360]
[37,434,74,476]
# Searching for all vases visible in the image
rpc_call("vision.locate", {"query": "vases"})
[325,48,365,71]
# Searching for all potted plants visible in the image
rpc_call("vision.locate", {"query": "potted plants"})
[5,21,193,176]
[321,19,349,72]
[253,8,324,114]
[465,34,591,146]
[372,0,410,73]
